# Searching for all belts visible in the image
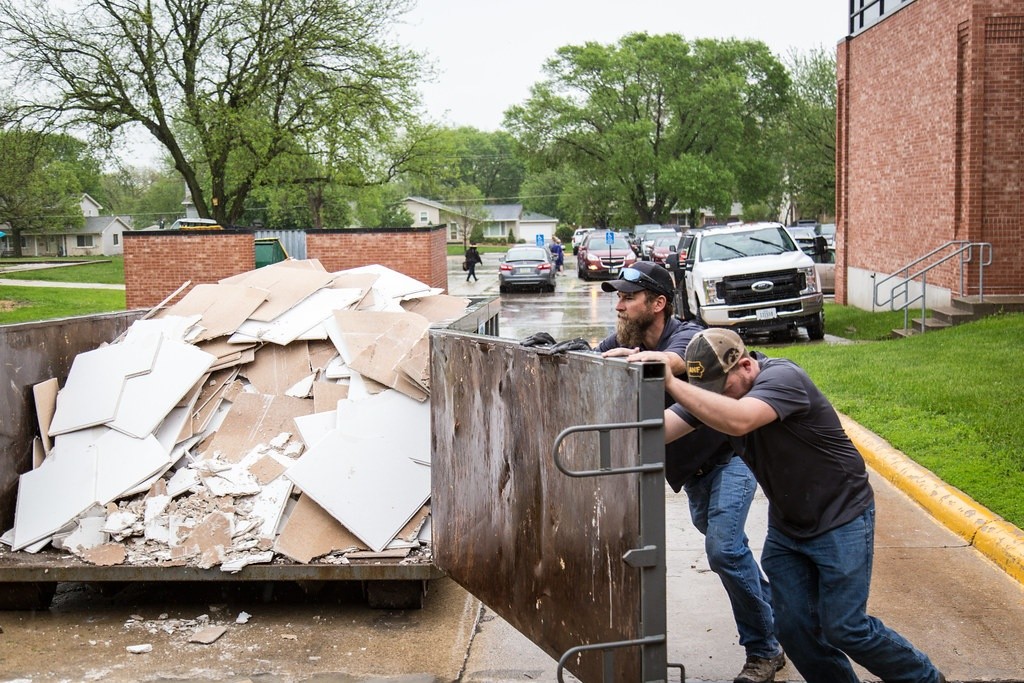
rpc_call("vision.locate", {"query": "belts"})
[694,464,714,477]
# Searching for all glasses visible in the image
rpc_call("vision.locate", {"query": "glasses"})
[617,267,675,295]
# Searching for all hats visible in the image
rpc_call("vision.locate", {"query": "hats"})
[601,261,675,303]
[684,328,744,395]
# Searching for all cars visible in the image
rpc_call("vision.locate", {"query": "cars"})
[515,222,836,281]
[499,247,556,292]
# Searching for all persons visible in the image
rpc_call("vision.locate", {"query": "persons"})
[604,327,949,683]
[811,226,828,264]
[463,242,483,281]
[516,259,787,683]
[548,236,566,277]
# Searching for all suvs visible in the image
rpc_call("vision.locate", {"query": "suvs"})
[665,221,826,339]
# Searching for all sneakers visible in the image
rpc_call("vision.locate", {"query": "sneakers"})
[734,642,787,683]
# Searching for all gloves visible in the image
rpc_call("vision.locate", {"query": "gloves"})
[519,332,558,347]
[548,337,593,358]
